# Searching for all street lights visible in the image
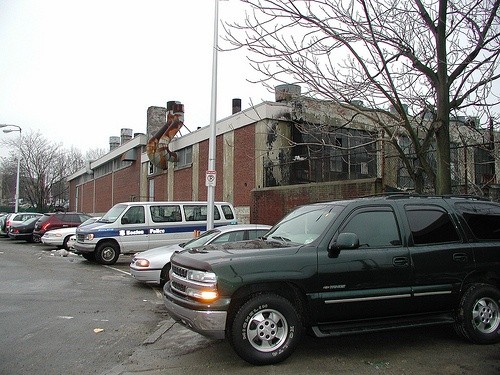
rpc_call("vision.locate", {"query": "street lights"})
[0,123,22,213]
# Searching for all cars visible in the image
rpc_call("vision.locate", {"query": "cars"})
[33,213,93,244]
[40,227,78,251]
[0,213,102,252]
[129,224,276,287]
[8,217,42,243]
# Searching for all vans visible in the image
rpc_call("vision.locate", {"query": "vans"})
[76,202,237,264]
[162,200,500,365]
